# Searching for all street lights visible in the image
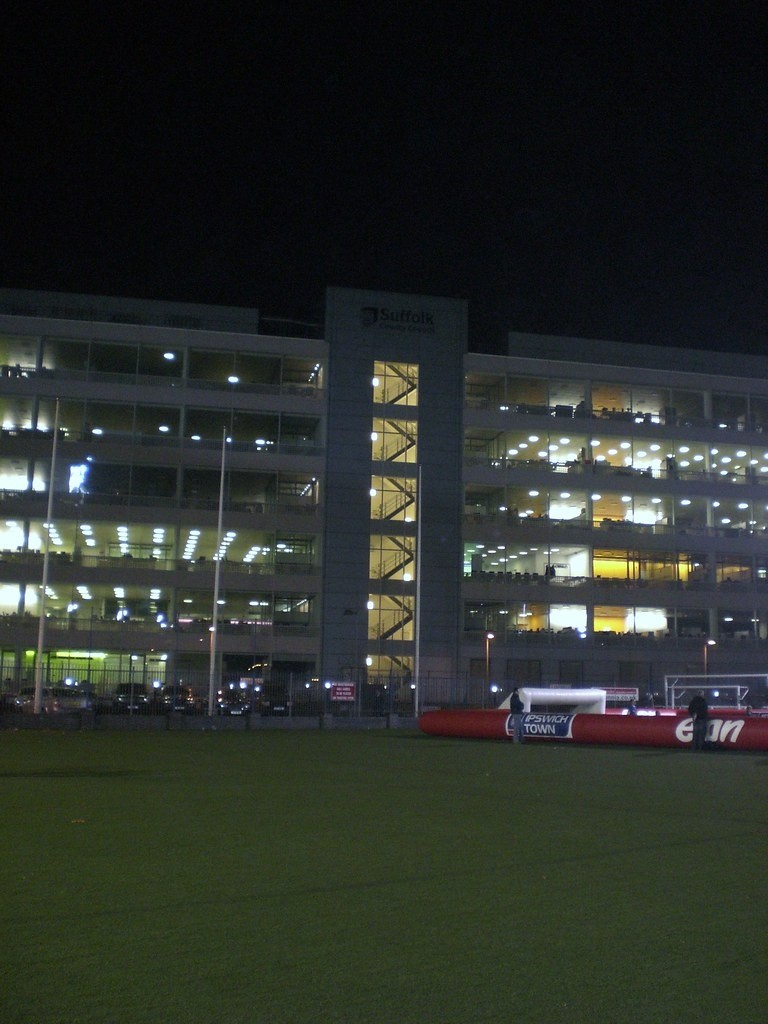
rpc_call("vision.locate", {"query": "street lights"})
[486,634,494,708]
[704,640,716,703]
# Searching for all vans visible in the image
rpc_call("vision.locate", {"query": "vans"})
[247,683,293,716]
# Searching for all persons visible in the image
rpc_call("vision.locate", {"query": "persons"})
[510,687,524,744]
[688,690,709,752]
[627,698,638,715]
[745,707,752,716]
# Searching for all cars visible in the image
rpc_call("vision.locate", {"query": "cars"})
[203,688,252,720]
[14,686,47,711]
[184,689,231,715]
[112,683,150,714]
[156,686,195,715]
[42,687,94,716]
